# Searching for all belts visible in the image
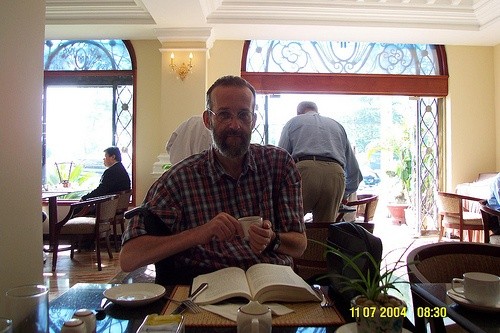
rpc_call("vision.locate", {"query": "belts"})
[294,155,344,169]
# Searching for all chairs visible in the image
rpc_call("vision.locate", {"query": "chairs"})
[479,198,500,244]
[347,194,378,224]
[52,196,120,271]
[295,222,374,277]
[77,189,133,250]
[436,192,486,242]
[408,242,500,332]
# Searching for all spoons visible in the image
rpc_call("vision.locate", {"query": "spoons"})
[95,301,113,312]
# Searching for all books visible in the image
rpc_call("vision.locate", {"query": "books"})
[189,263,321,304]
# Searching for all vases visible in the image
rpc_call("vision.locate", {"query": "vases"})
[388,205,407,226]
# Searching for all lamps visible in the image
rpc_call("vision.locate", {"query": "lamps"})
[167,52,194,80]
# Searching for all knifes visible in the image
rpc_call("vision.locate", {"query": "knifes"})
[171,283,208,314]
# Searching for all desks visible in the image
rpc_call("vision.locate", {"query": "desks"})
[410,283,500,333]
[42,183,88,251]
[16,283,417,333]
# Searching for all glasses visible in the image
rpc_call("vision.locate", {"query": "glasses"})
[208,109,254,119]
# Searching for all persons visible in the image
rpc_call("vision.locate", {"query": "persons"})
[165,114,214,166]
[487,173,500,212]
[119,76,306,286]
[73,146,130,250]
[278,101,363,223]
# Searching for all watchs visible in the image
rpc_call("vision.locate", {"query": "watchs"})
[268,232,280,251]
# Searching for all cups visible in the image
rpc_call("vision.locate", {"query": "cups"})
[236,305,272,333]
[5,284,49,333]
[447,272,500,306]
[237,216,264,241]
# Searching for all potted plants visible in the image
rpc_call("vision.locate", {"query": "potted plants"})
[304,240,417,333]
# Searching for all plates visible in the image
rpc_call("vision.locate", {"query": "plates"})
[103,283,166,307]
[447,287,500,308]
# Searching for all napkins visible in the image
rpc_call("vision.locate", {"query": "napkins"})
[200,301,294,321]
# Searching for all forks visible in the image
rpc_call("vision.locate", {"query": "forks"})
[164,295,202,314]
